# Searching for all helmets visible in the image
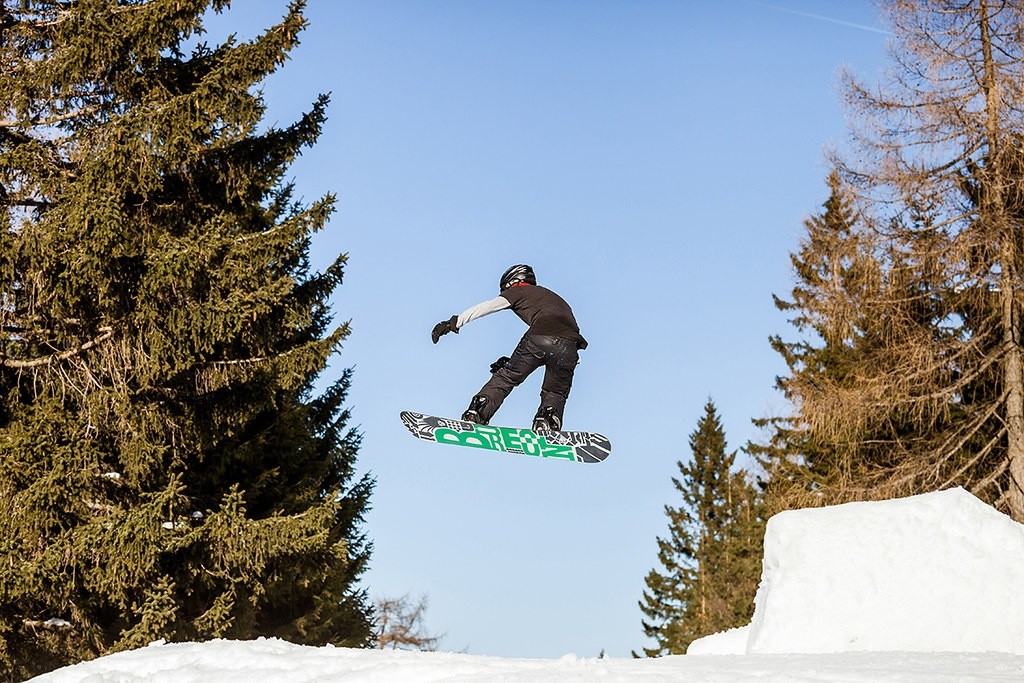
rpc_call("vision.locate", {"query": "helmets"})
[500,264,537,290]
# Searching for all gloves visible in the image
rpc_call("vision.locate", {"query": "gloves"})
[489,356,510,373]
[431,315,460,343]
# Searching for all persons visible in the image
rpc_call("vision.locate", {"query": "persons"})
[431,264,588,431]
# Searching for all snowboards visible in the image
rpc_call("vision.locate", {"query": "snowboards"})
[400,411,612,463]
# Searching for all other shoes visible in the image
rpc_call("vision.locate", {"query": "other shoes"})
[533,406,561,430]
[461,394,488,424]
